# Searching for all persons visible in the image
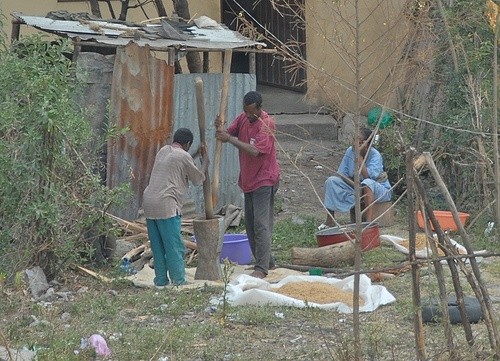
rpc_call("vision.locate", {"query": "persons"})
[213,91,279,281]
[315,127,392,230]
[142,128,210,286]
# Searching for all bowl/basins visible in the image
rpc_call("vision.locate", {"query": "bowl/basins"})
[414,210,470,231]
[191,233,253,265]
[315,222,380,252]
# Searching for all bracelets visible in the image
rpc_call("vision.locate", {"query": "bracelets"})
[229,134,232,143]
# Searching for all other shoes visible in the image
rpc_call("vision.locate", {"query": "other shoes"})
[314,224,331,232]
